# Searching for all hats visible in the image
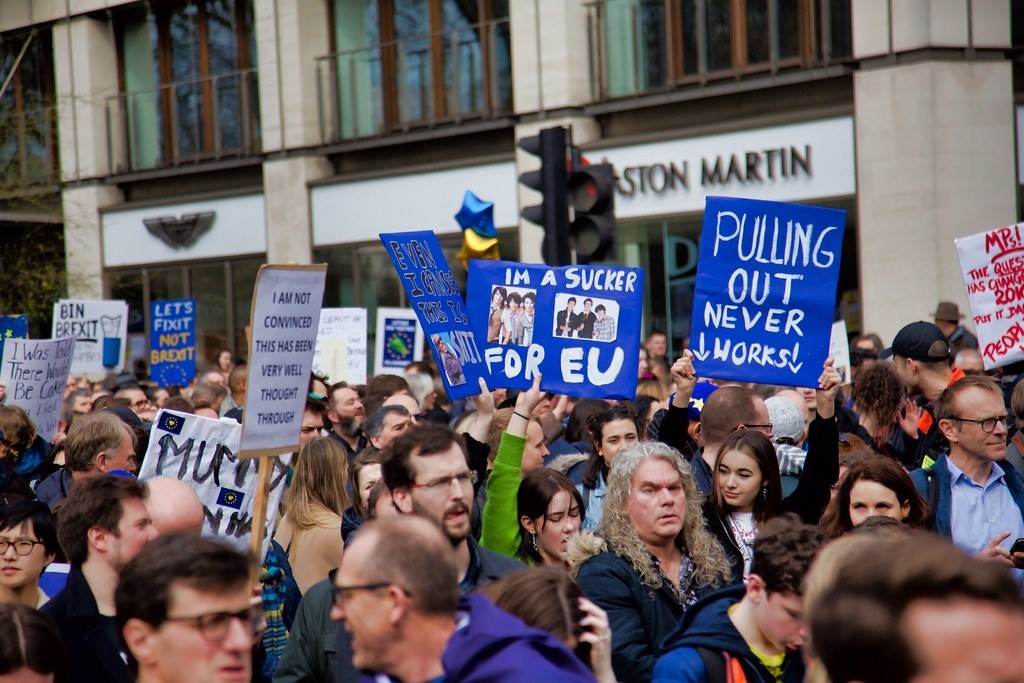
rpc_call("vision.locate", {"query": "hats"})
[930,302,966,320]
[669,382,719,419]
[498,389,555,409]
[117,369,139,389]
[103,406,153,431]
[880,320,951,362]
[764,396,805,443]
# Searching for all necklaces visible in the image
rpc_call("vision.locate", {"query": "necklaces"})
[730,515,752,561]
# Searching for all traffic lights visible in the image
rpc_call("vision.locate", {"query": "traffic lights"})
[516,126,573,267]
[563,162,618,263]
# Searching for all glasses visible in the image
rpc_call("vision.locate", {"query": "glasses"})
[406,470,478,495]
[947,415,1014,432]
[165,606,263,642]
[131,400,152,410]
[330,582,412,610]
[839,432,851,449]
[731,423,773,434]
[0,540,44,556]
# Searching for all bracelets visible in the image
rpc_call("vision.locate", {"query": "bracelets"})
[513,412,530,421]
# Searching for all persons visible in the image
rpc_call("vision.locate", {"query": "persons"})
[0,303,1024,683]
[575,298,597,339]
[487,287,508,343]
[593,305,616,341]
[432,334,466,385]
[517,291,536,345]
[556,297,580,337]
[500,293,525,345]
